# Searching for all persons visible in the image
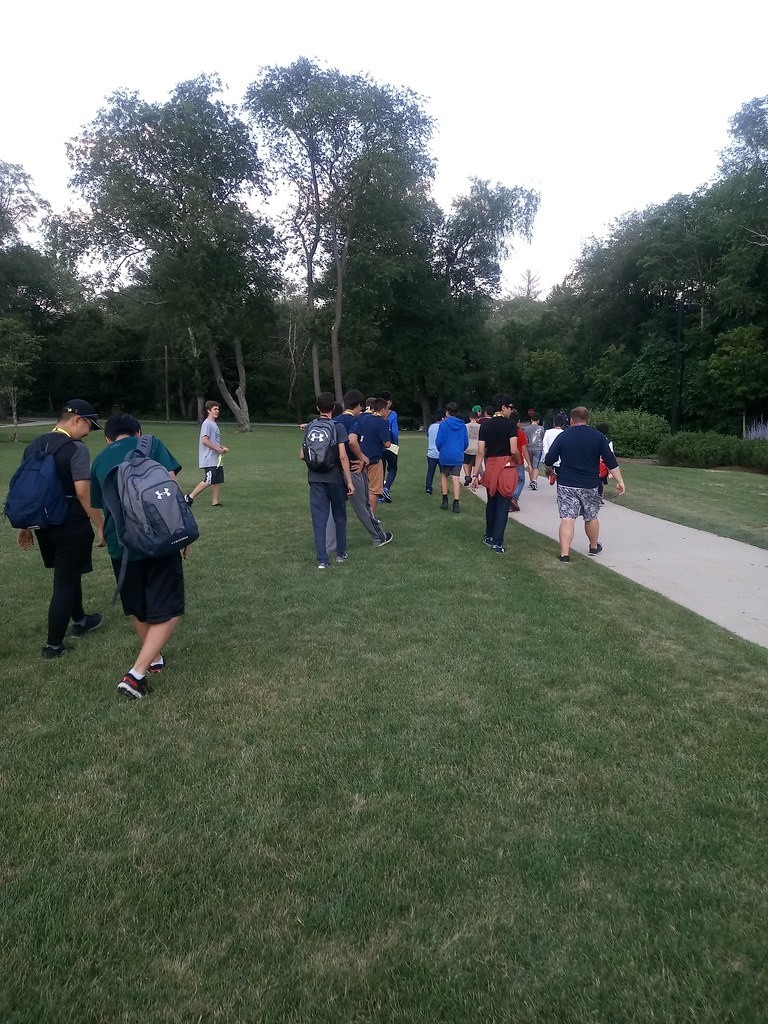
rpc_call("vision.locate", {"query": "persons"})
[18,399,104,659]
[435,402,469,513]
[470,393,521,554]
[426,409,614,506]
[299,391,399,518]
[90,414,190,700]
[544,406,626,564]
[326,390,393,556]
[301,392,355,570]
[184,400,228,508]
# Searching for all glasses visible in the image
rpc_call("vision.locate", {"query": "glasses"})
[80,416,94,432]
[507,406,514,411]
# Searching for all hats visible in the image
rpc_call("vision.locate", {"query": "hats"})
[63,399,103,431]
[472,405,482,412]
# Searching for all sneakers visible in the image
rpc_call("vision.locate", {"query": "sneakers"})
[336,553,348,562]
[483,536,493,546]
[40,643,72,659]
[557,554,570,563]
[319,562,329,569]
[589,542,602,557]
[213,502,223,506]
[149,656,164,673]
[491,545,505,552]
[382,487,393,503]
[184,494,194,508]
[376,531,393,545]
[118,674,150,699]
[71,613,103,637]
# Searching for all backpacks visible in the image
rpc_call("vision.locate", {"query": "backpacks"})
[5,435,84,528]
[303,415,340,473]
[102,435,200,558]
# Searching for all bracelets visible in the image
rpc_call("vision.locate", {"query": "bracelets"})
[472,475,478,477]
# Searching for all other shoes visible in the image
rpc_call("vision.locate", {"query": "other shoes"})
[426,476,539,513]
